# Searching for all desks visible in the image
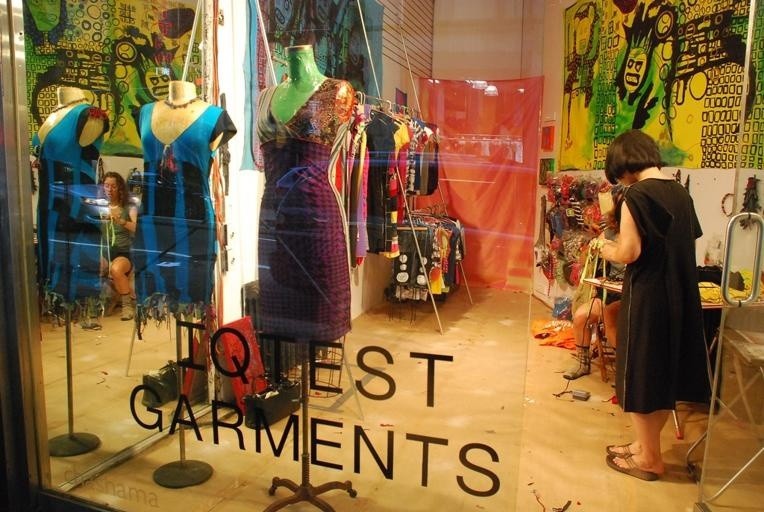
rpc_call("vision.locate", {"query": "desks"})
[584,278,764,442]
[686,326,764,505]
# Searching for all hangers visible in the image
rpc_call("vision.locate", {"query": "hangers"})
[403,200,449,228]
[351,90,422,127]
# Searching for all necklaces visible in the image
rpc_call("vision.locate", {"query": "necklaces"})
[161,99,202,110]
[48,98,88,113]
[107,204,119,210]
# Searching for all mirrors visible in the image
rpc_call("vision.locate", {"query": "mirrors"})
[21,2,212,491]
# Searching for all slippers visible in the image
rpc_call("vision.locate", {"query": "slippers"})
[606,442,656,480]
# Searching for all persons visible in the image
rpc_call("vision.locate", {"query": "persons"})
[100,172,137,321]
[136,80,237,328]
[563,188,627,388]
[602,129,708,481]
[30,87,109,326]
[254,43,357,342]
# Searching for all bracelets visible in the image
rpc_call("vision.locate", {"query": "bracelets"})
[122,219,127,226]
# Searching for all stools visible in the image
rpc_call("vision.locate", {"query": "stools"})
[103,271,137,315]
[587,288,616,383]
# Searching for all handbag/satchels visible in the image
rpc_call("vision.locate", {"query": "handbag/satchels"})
[142,363,178,408]
[243,372,300,430]
